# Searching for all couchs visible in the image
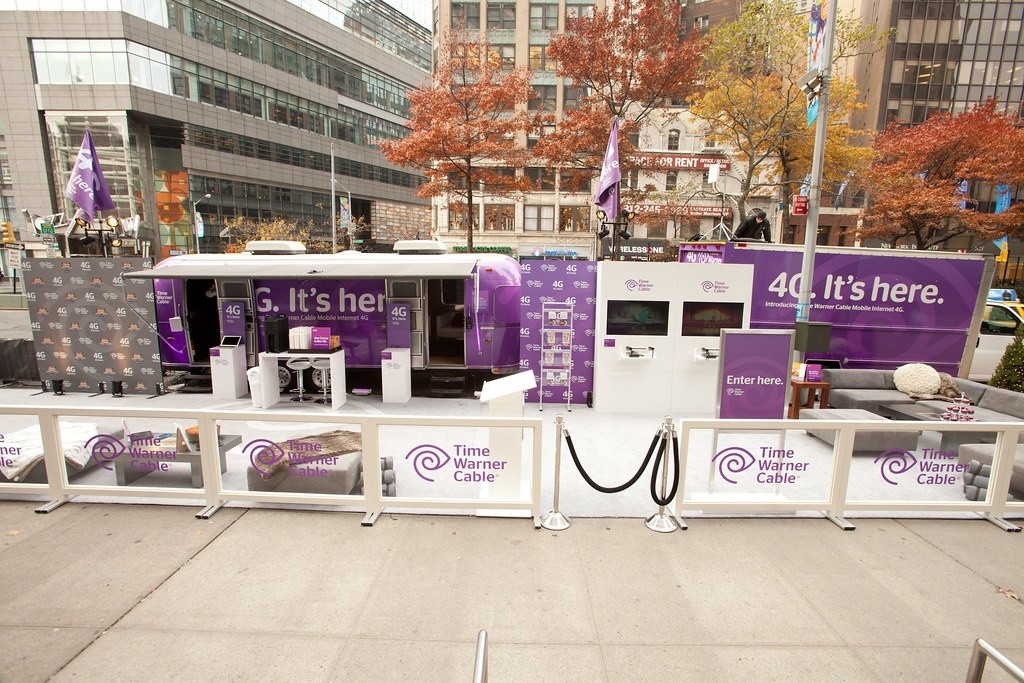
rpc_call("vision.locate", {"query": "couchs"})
[823,369,1024,502]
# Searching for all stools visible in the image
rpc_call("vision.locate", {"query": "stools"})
[799,409,919,459]
[287,355,314,402]
[310,357,332,405]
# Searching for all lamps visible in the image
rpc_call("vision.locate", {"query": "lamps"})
[51,379,66,396]
[111,380,126,397]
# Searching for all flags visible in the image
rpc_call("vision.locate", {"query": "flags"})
[593,116,621,220]
[63,132,117,224]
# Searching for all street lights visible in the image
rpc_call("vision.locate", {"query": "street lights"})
[75,216,122,258]
[193,194,211,254]
[596,209,635,261]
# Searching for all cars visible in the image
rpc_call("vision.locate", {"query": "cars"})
[968,288,1024,382]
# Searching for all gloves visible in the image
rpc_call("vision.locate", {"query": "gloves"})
[730,238,737,242]
[765,238,771,243]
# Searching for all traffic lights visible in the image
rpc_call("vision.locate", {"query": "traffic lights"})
[1,222,15,242]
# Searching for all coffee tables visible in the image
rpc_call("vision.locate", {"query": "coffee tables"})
[878,404,997,457]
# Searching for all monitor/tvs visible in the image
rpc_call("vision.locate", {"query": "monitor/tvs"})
[680,301,744,337]
[606,300,669,337]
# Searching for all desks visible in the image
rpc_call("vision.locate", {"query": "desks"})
[94,432,243,488]
[258,351,347,410]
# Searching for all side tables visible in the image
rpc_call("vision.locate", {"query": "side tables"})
[788,378,830,419]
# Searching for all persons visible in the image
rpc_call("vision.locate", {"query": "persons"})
[708,310,717,329]
[192,281,220,362]
[729,211,772,243]
[631,305,654,330]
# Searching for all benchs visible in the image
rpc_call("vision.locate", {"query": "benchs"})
[0,422,125,483]
[248,433,362,496]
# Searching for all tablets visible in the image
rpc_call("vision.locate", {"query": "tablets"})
[221,335,243,347]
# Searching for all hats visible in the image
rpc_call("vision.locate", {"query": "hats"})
[758,213,766,221]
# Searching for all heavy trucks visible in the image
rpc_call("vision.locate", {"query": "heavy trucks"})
[120,240,521,389]
